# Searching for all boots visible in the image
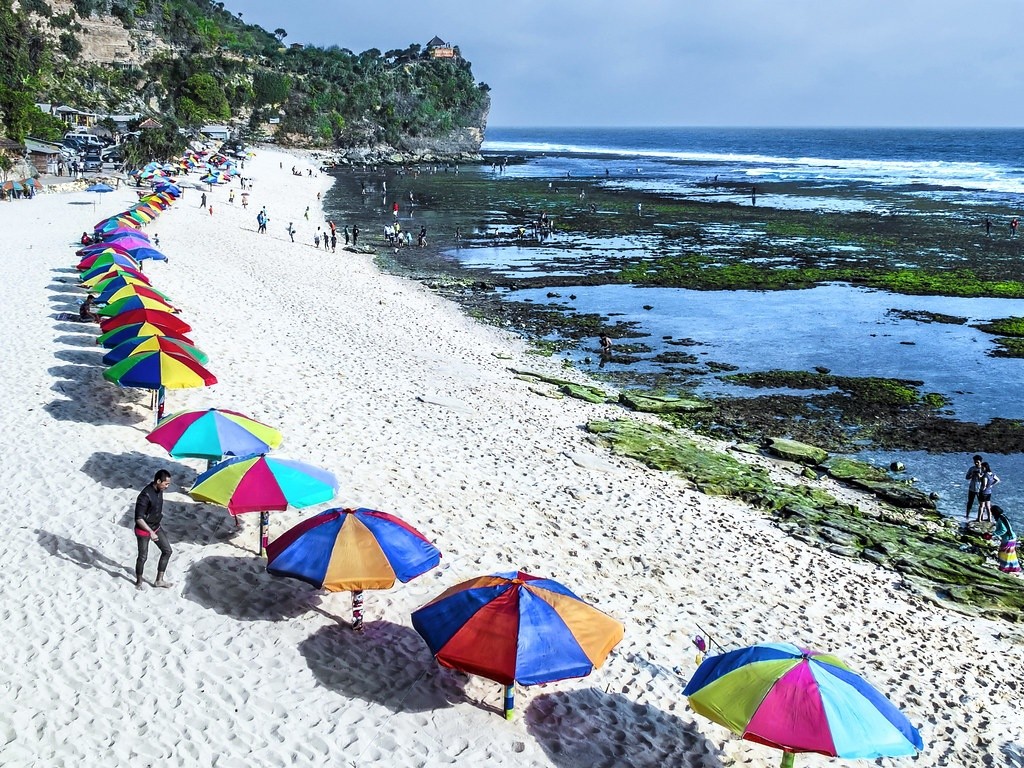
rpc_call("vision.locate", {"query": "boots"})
[154,572,173,588]
[136,574,143,587]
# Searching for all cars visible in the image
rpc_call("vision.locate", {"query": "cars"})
[57,133,123,171]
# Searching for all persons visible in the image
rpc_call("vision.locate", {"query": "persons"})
[256,150,1024,356]
[984,505,1022,576]
[134,469,174,590]
[977,462,1000,523]
[965,455,986,521]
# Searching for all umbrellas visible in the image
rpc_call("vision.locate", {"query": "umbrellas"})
[411,569,626,721]
[264,505,443,633]
[146,407,283,473]
[0,140,254,422]
[681,643,923,768]
[187,453,339,559]
